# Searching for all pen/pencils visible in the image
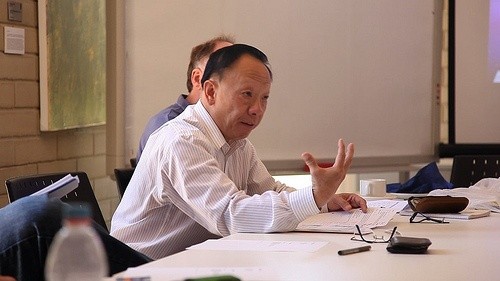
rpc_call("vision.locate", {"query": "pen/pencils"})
[338,245,371,255]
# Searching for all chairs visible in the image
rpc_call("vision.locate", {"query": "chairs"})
[449,155,500,189]
[5,158,137,281]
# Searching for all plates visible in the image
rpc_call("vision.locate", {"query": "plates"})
[358,194,398,200]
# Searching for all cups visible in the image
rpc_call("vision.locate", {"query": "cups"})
[360,179,386,197]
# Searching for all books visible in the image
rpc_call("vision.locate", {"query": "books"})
[400,210,491,219]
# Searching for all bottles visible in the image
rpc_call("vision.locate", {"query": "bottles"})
[44,203,107,281]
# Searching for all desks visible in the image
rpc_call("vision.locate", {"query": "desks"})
[111,187,500,281]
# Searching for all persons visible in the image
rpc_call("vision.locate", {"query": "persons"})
[108,44,367,264]
[136,34,237,168]
[0,193,153,281]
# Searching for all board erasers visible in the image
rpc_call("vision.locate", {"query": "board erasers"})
[305,163,337,172]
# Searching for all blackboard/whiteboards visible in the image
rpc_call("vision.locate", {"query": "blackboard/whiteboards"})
[105,0,440,179]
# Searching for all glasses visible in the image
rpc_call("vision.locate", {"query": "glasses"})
[351,224,402,244]
